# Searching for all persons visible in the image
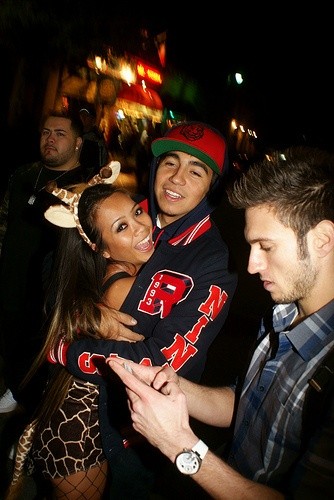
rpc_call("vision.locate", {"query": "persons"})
[28,118,239,500]
[20,182,157,499]
[105,143,334,500]
[0,111,102,413]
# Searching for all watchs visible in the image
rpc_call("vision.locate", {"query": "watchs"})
[175,439,209,476]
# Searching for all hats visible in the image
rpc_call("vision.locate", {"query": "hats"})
[151,119,226,176]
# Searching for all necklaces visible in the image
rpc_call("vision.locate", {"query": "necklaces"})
[25,160,76,207]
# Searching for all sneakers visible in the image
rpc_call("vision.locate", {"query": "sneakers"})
[0,388,27,414]
